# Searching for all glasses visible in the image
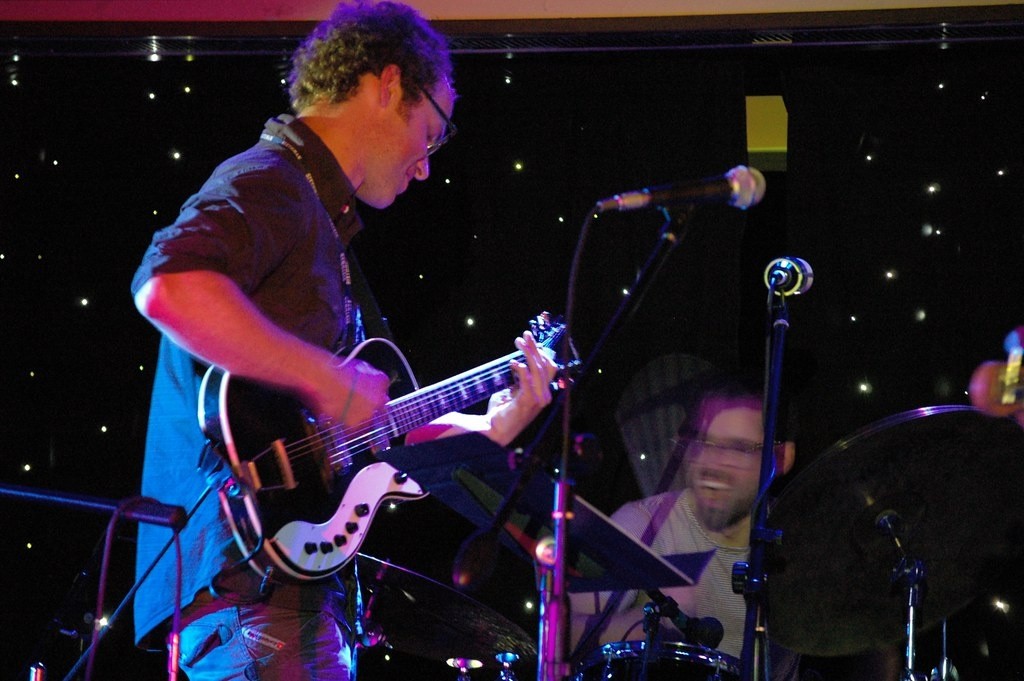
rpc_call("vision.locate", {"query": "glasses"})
[415,80,465,153]
[679,434,762,469]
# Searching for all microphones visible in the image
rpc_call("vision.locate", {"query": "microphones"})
[643,589,724,650]
[597,165,766,214]
[766,257,814,296]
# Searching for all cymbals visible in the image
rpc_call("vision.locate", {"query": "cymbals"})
[345,546,537,664]
[752,405,1024,660]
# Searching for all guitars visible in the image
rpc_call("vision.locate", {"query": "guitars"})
[196,312,586,580]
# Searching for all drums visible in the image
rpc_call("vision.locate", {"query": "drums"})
[570,640,745,681]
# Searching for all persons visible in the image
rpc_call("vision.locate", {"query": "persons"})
[568,392,801,681]
[132,0,558,681]
